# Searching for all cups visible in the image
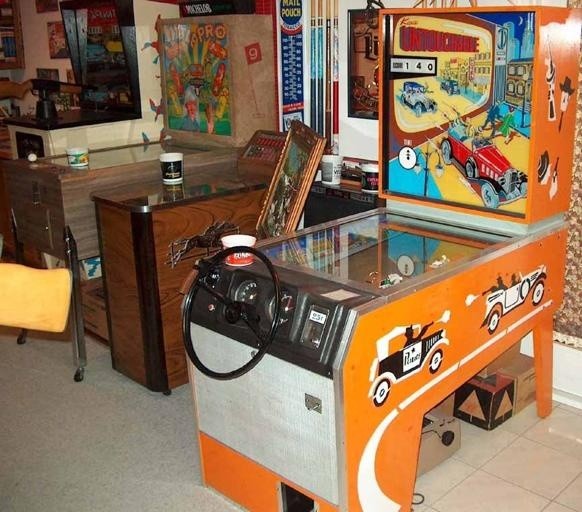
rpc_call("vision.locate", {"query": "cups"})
[320,154,344,186]
[160,152,185,187]
[361,164,378,194]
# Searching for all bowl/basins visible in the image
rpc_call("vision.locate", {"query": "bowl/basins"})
[220,234,257,266]
[67,147,90,167]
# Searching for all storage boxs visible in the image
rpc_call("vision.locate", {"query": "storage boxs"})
[498,353,537,416]
[477,340,521,379]
[453,373,515,432]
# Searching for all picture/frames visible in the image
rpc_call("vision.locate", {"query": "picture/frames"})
[0,0,26,70]
[346,7,379,120]
[255,119,329,242]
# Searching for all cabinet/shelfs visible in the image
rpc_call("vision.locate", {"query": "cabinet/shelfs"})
[90,166,272,395]
